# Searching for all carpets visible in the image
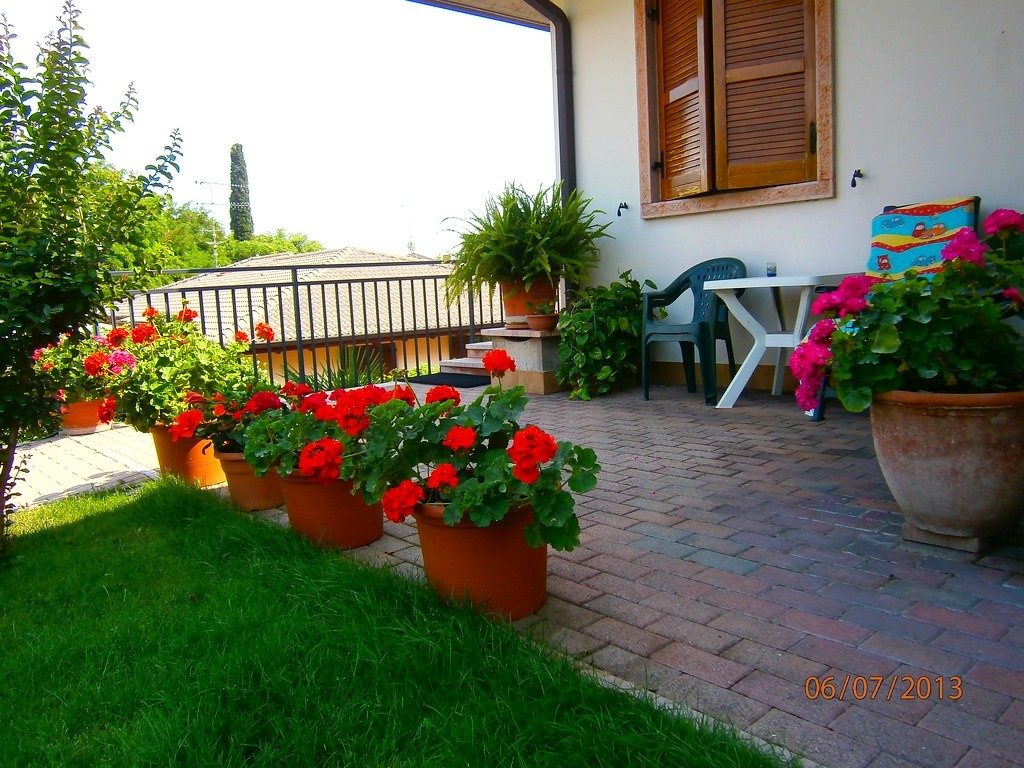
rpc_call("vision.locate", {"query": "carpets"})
[399,373,491,388]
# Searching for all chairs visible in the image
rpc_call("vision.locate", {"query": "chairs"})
[641,258,748,406]
[806,195,983,423]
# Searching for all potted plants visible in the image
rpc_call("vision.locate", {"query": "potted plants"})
[524,291,560,332]
[439,178,617,328]
[555,269,669,401]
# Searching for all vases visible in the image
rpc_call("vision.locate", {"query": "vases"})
[274,464,383,551]
[868,390,1024,537]
[410,470,547,622]
[214,446,283,512]
[58,399,112,435]
[149,417,226,486]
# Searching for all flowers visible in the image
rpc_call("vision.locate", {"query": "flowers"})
[790,209,1024,412]
[33,296,601,552]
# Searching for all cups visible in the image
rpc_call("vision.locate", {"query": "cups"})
[767,262,777,277]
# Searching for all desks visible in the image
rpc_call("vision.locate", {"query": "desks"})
[702,271,867,408]
